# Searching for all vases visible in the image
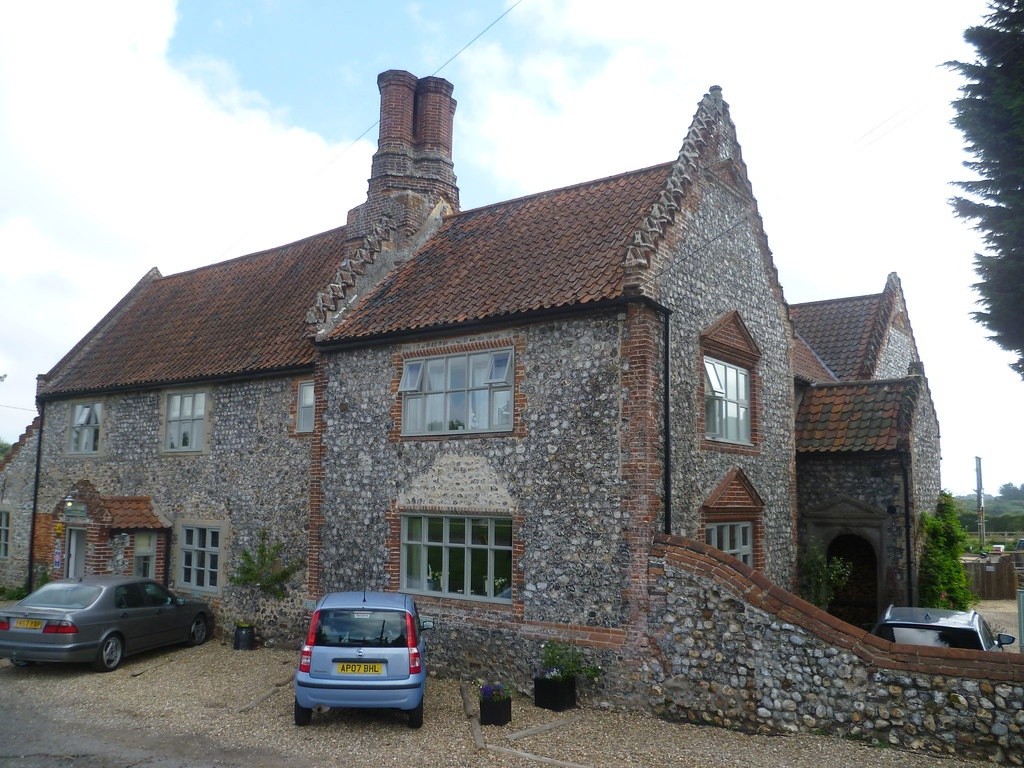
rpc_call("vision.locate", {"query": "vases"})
[480,697,511,725]
[535,676,576,711]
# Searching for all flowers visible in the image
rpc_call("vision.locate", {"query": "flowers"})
[534,640,606,683]
[431,570,443,591]
[470,677,514,702]
[495,578,508,595]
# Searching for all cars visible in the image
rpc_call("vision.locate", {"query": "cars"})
[0,574,214,674]
[294,591,434,729]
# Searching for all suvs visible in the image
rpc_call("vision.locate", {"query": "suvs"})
[872,603,1016,655]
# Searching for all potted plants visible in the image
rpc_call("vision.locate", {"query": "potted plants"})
[233,530,306,650]
